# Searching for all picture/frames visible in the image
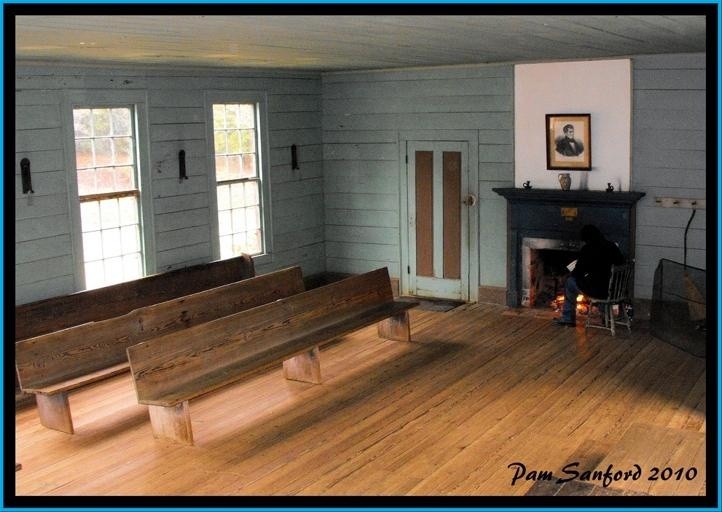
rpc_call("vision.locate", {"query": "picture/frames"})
[545,113,592,172]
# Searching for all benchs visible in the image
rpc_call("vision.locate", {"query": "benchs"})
[16,254,419,446]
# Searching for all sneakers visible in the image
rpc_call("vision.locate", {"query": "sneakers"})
[552,319,576,327]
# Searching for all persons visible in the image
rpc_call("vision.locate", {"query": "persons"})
[556,125,581,156]
[553,224,627,327]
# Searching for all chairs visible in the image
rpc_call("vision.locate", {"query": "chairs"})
[583,259,634,337]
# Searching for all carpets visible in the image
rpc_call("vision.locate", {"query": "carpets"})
[393,293,466,313]
